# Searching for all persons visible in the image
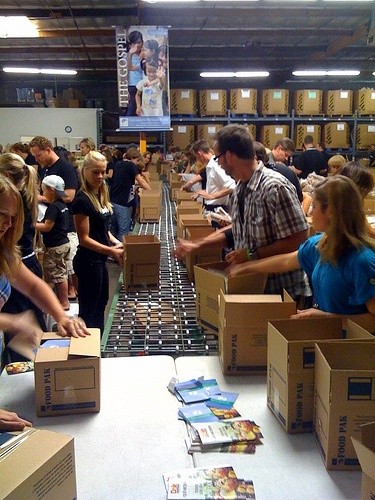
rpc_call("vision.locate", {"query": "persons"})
[152,124,375,262]
[71,150,125,339]
[117,30,169,116]
[0,135,151,311]
[0,152,47,362]
[0,174,92,433]
[172,124,312,313]
[231,175,375,338]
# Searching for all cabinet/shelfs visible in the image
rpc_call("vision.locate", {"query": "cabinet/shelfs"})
[159,113,375,164]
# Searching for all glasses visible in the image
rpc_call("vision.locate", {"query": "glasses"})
[213,151,228,163]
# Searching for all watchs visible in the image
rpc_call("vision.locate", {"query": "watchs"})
[249,248,257,260]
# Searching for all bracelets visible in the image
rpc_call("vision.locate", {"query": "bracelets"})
[247,248,250,261]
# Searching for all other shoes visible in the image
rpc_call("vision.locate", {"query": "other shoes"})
[68,296,77,302]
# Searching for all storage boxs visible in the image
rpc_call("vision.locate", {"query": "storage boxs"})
[122,154,375,500]
[0,426,78,500]
[7,328,101,417]
[51,87,83,108]
[167,88,375,150]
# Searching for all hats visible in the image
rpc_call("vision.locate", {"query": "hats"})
[42,175,68,198]
